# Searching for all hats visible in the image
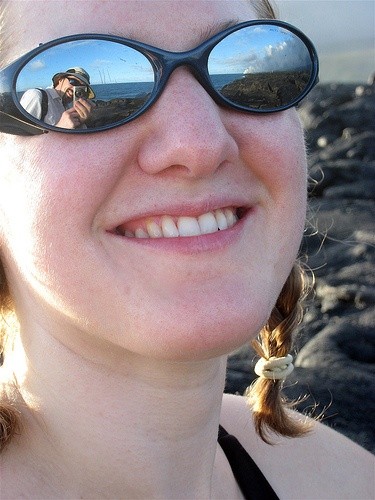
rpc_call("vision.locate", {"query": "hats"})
[52,66,96,99]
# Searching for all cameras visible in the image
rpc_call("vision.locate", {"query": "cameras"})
[73,86,88,105]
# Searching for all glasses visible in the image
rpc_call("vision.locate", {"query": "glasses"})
[66,77,86,86]
[0,18,320,137]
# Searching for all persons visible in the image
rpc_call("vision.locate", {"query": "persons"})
[19,66,97,130]
[0,1,375,500]
